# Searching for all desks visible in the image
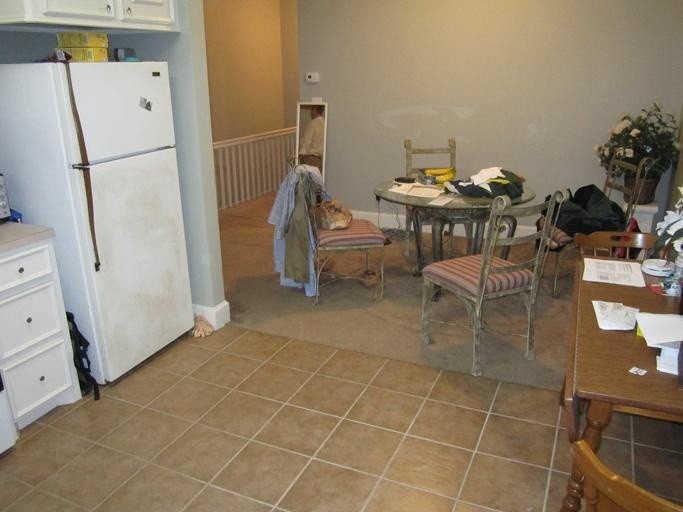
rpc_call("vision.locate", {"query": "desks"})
[559,254,683,512]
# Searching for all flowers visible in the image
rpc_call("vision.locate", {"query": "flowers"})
[592,98,682,177]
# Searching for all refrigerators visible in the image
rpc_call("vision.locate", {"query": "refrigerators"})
[0,59,196,385]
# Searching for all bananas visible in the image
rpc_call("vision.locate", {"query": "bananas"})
[424,165,457,188]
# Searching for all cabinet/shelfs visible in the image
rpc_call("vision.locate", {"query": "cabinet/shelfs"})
[0,244,74,421]
[42,0,175,23]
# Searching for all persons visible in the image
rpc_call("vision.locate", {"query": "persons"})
[299,105,324,173]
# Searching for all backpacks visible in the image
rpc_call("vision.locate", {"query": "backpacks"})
[443,167,525,198]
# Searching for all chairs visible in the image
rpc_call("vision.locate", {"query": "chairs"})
[539,150,658,301]
[421,189,566,375]
[404,136,517,306]
[298,167,391,308]
[572,440,683,511]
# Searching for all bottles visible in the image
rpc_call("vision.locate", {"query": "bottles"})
[674,244,683,275]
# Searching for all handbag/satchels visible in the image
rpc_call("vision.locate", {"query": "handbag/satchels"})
[66,311,101,401]
[308,199,352,230]
[535,181,627,251]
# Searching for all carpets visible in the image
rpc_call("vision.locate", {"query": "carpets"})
[213,192,580,390]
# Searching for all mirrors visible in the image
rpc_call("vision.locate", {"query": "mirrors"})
[295,102,328,183]
